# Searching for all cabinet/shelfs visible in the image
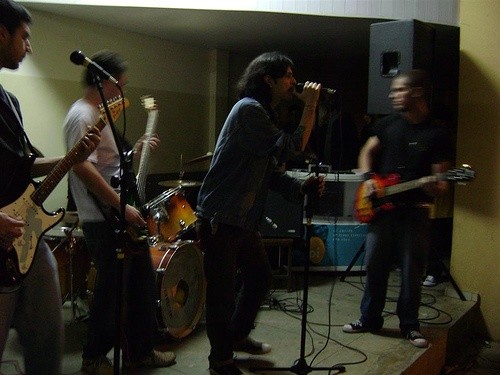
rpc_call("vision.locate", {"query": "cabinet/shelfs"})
[285,170,369,272]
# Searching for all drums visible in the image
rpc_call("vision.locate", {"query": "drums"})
[40,178,204,347]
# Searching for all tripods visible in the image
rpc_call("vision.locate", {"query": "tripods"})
[63,237,89,324]
[247,103,346,375]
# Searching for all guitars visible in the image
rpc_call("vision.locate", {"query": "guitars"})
[354,161,476,224]
[0,95,130,278]
[110,93,161,241]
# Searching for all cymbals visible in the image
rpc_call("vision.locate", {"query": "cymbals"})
[42,211,80,225]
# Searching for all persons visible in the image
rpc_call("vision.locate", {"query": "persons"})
[341,72,452,347]
[0,1,176,375]
[196,53,321,375]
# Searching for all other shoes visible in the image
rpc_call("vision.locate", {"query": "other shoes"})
[422,275,437,286]
[82,358,113,375]
[209,366,242,375]
[124,349,176,369]
[232,337,272,355]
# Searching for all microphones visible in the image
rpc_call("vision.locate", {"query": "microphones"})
[70,51,120,89]
[184,152,213,165]
[295,83,338,95]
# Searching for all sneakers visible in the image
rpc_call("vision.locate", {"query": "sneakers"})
[339,320,385,332]
[401,329,429,348]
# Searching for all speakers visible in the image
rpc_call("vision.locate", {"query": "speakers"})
[369,19,461,116]
